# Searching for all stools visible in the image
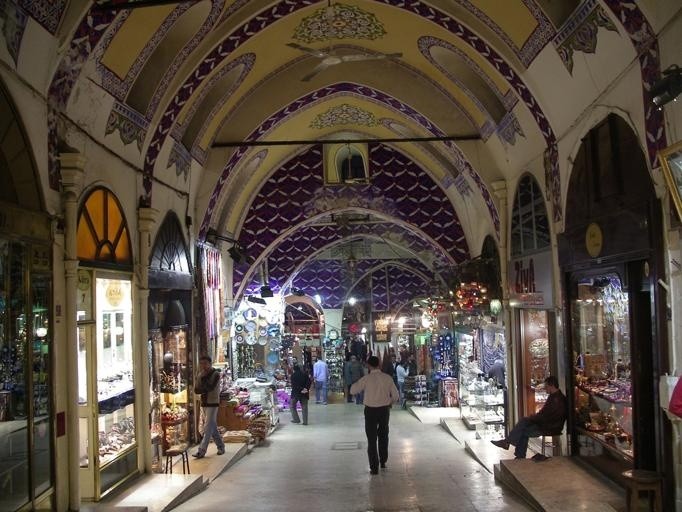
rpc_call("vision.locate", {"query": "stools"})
[165,443,191,475]
[540,433,564,459]
[622,470,666,512]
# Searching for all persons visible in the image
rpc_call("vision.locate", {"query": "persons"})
[489,357,505,393]
[490,377,567,460]
[193,356,225,458]
[312,355,329,405]
[351,356,363,405]
[350,357,399,474]
[617,358,626,379]
[289,366,312,424]
[389,355,400,384]
[345,356,355,401]
[668,376,682,417]
[343,336,371,355]
[575,351,583,371]
[402,353,415,377]
[293,357,303,371]
[396,362,409,407]
[466,356,478,373]
[399,344,408,362]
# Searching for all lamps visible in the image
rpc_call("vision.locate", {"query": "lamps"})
[348,295,357,307]
[313,292,321,304]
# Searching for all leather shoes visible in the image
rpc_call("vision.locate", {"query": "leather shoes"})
[491,439,510,450]
[191,451,205,459]
[217,446,225,455]
